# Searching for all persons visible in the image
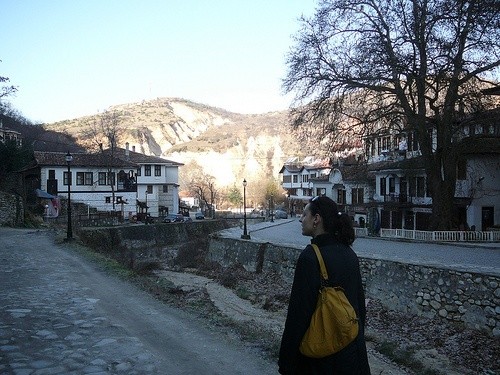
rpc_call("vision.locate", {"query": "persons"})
[277,196,371,375]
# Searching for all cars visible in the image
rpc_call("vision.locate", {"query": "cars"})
[196,212,204,220]
[163,214,192,223]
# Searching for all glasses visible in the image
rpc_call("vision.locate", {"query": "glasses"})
[312,195,326,213]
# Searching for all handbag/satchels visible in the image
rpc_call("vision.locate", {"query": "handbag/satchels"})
[298,243,360,357]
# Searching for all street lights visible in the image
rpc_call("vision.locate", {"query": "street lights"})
[241,178,250,239]
[64,150,76,241]
[270,193,274,223]
